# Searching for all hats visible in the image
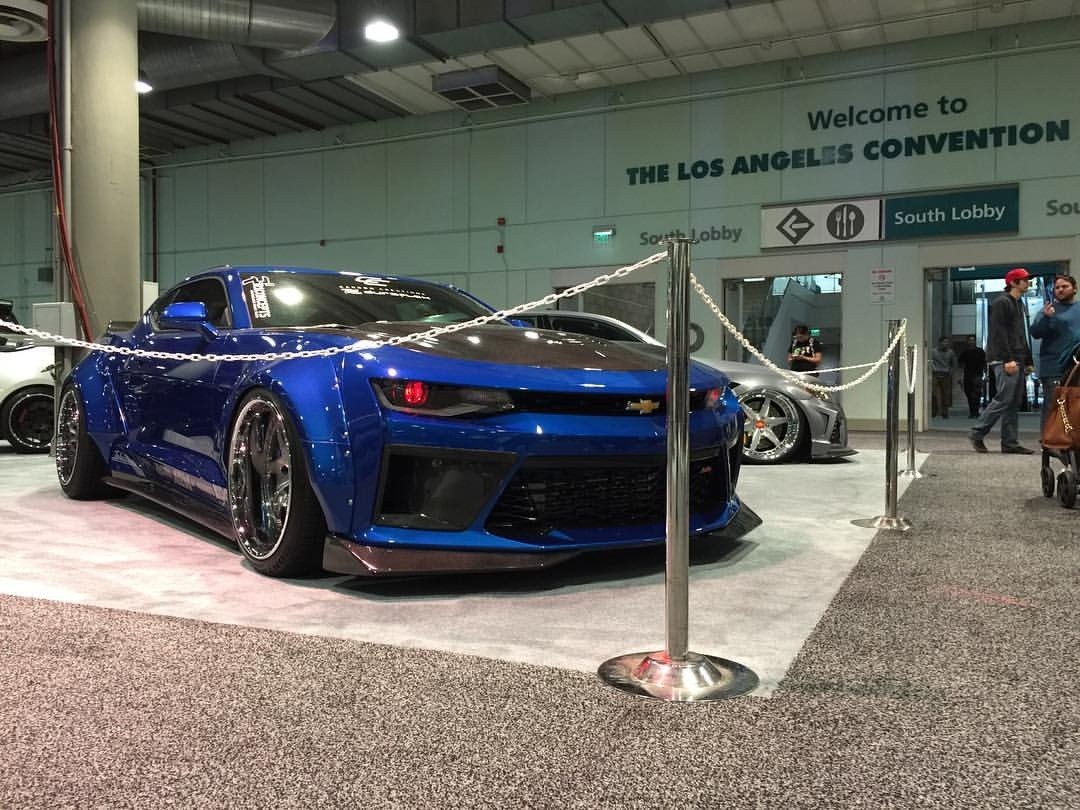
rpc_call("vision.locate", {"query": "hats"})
[1006,269,1036,283]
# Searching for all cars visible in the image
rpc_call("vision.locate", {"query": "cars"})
[413,310,860,466]
[53,265,748,576]
[0,298,55,454]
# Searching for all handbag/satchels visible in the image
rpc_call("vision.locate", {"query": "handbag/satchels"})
[1039,362,1080,450]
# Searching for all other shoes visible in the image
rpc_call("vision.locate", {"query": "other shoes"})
[1001,445,1034,454]
[968,436,988,452]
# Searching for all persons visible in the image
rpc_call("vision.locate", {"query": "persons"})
[785,324,824,378]
[959,335,987,419]
[931,336,957,418]
[1029,275,1079,450]
[966,267,1036,456]
